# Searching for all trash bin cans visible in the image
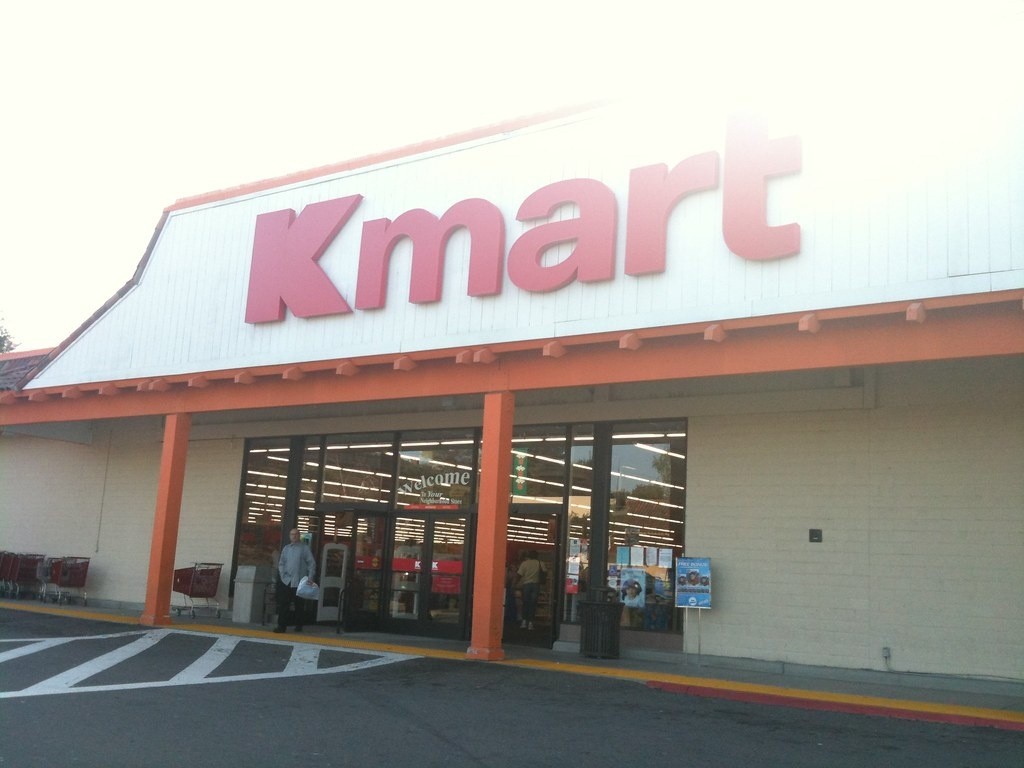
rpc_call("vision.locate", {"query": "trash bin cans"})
[232,564,273,625]
[576,599,624,658]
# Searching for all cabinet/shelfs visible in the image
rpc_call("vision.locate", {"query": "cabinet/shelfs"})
[533,591,551,618]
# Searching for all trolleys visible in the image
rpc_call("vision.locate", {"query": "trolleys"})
[0,551,47,604]
[50,557,90,605]
[173,562,224,619]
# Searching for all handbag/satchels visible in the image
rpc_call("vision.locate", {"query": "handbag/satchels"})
[539,560,546,584]
[296,576,320,601]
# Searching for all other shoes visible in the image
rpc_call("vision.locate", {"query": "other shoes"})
[528,626,535,630]
[519,624,527,628]
[273,625,286,633]
[295,626,303,632]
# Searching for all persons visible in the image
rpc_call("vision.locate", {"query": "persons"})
[510,550,548,632]
[623,582,639,601]
[273,528,316,633]
